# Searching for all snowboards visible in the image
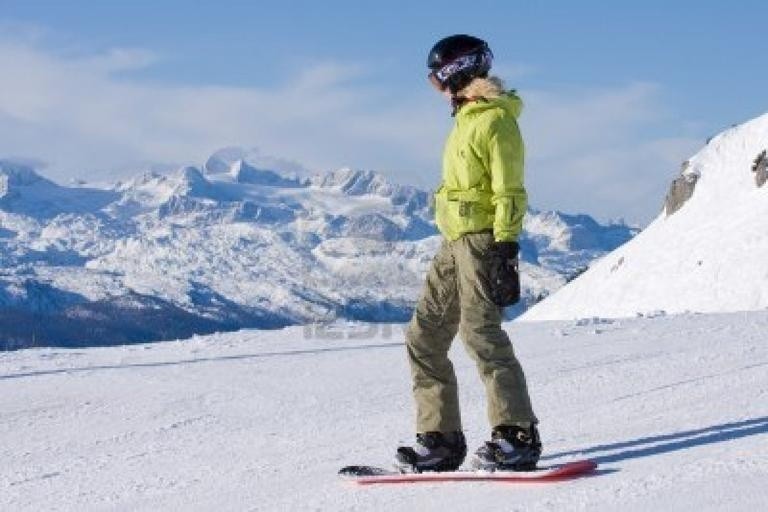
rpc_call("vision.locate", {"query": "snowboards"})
[338,458,598,485]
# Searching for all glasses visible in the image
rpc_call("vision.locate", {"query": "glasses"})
[428,56,492,92]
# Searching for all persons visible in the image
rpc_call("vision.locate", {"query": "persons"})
[393,33,542,472]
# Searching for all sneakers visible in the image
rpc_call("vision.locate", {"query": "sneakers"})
[396,432,466,471]
[474,425,543,471]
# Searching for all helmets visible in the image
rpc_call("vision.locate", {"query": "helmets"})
[427,34,492,71]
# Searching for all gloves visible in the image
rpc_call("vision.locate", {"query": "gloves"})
[483,242,520,307]
